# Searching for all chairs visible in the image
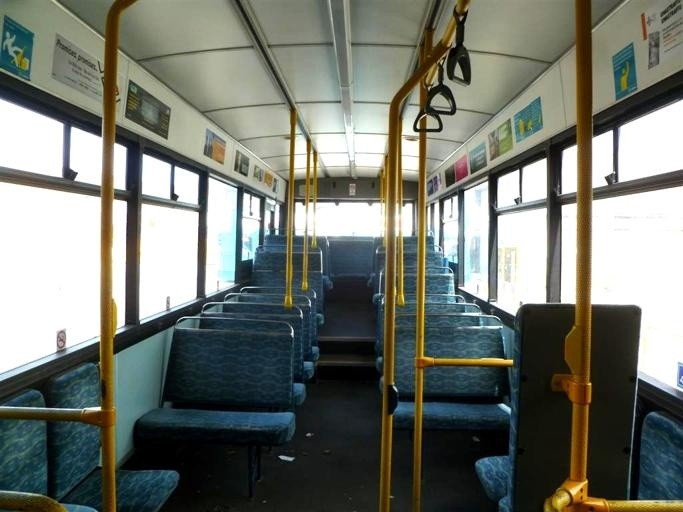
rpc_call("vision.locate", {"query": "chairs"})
[0,390,98,511]
[638,409,683,500]
[476,303,641,512]
[43,362,181,512]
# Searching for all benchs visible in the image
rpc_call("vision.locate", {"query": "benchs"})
[223,293,316,382]
[374,268,456,305]
[394,327,511,435]
[265,237,333,291]
[375,304,482,373]
[379,314,503,396]
[240,286,319,362]
[375,294,465,356]
[199,302,307,405]
[368,244,443,294]
[250,270,324,328]
[252,246,323,273]
[273,235,434,282]
[133,316,296,501]
